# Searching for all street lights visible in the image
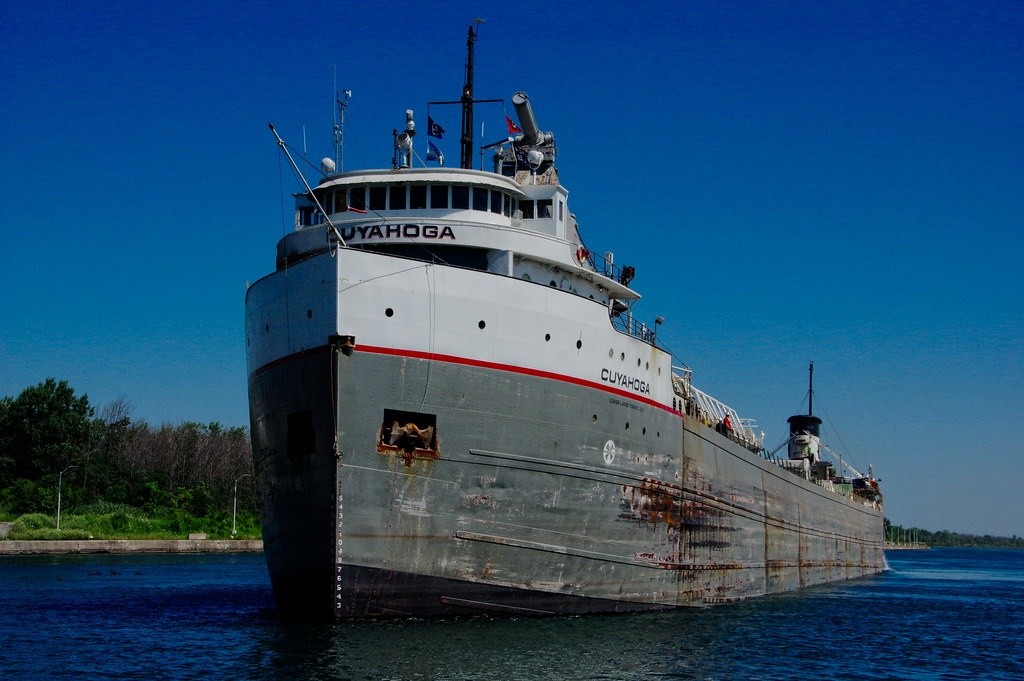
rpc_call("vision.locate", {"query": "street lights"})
[232,474,251,534]
[57,466,79,530]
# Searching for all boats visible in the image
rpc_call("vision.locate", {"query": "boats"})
[245,20,886,623]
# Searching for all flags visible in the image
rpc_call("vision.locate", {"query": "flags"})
[506,114,522,135]
[427,139,444,167]
[427,115,445,138]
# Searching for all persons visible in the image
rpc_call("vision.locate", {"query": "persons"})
[724,413,732,438]
[716,420,724,436]
[702,411,709,426]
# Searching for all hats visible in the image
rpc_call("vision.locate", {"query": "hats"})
[725,412,730,415]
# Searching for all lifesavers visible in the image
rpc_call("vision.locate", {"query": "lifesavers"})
[576,244,586,265]
